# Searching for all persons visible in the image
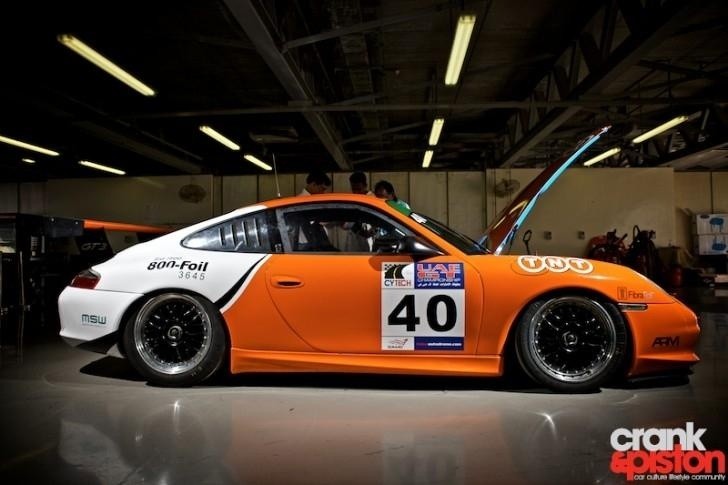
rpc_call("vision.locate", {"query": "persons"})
[372,179,413,253]
[294,170,333,248]
[340,171,376,252]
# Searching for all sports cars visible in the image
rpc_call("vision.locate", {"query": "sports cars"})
[57,122,703,392]
